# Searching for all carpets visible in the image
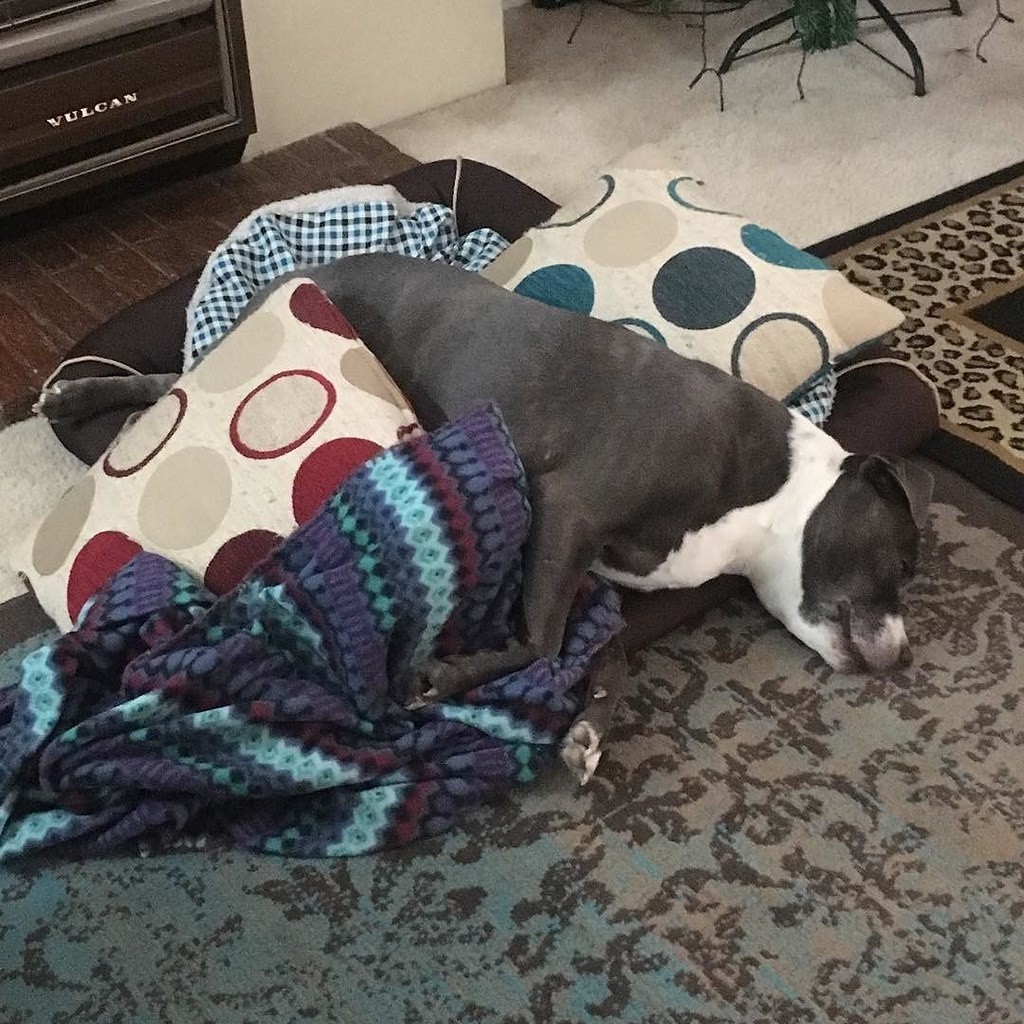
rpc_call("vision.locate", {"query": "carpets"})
[800,155,1024,522]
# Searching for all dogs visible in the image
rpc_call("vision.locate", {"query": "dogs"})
[29,252,936,787]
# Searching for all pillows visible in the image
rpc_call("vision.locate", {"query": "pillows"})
[479,144,903,405]
